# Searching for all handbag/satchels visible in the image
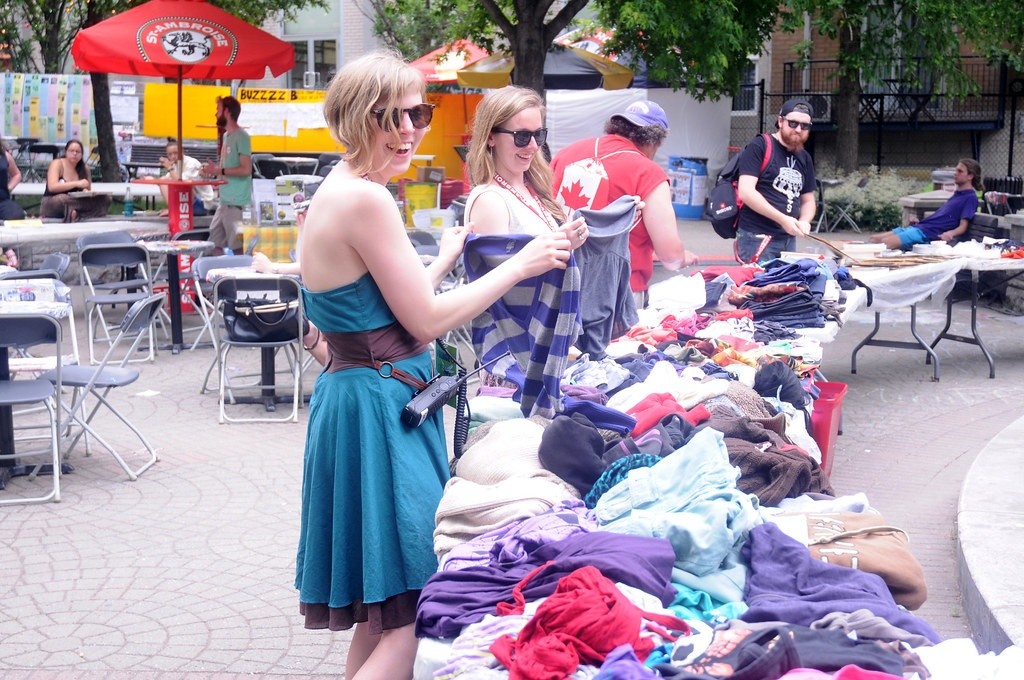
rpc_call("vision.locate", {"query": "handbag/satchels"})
[224,299,309,342]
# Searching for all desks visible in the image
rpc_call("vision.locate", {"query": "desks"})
[276,175,324,186]
[35,142,67,157]
[413,252,1024,680]
[137,240,216,355]
[207,267,313,412]
[132,178,229,313]
[273,157,320,175]
[822,179,844,193]
[0,219,170,309]
[234,224,299,263]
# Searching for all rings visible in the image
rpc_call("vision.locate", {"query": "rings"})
[579,235,584,240]
[577,228,581,234]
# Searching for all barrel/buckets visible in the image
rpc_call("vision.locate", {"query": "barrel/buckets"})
[669,157,709,218]
[404,182,436,228]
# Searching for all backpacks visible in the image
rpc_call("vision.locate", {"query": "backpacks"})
[556,138,640,209]
[703,134,774,239]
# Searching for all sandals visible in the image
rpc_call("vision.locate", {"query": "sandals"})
[6,255,18,268]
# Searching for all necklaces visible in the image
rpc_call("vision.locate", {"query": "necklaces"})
[359,169,373,182]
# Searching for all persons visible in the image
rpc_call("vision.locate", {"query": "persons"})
[869,158,982,252]
[199,95,254,255]
[40,139,112,223]
[461,85,645,387]
[0,137,26,221]
[732,94,817,268]
[157,141,214,218]
[550,99,702,307]
[251,175,332,366]
[296,53,572,680]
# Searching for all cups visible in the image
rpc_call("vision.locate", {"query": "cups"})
[412,209,456,231]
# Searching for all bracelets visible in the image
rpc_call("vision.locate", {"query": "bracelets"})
[222,168,225,175]
[168,168,174,172]
[272,262,279,273]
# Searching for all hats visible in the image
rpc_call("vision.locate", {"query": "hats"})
[611,100,668,129]
[960,158,984,192]
[775,99,813,129]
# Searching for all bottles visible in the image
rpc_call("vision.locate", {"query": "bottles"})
[123,187,134,218]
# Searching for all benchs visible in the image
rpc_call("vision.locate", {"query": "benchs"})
[121,145,220,183]
[9,182,215,227]
[904,214,999,303]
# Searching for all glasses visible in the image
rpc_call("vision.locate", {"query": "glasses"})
[782,115,812,130]
[370,103,435,132]
[491,126,547,147]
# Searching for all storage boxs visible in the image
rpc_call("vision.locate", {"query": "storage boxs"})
[810,382,849,477]
[386,166,464,223]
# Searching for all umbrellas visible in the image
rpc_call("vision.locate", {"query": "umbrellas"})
[409,33,498,85]
[71,0,296,181]
[557,22,713,90]
[458,37,633,91]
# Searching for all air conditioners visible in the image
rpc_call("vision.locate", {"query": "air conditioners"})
[792,92,831,123]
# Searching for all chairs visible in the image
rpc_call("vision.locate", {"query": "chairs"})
[0,137,344,506]
[811,178,828,234]
[830,178,869,233]
